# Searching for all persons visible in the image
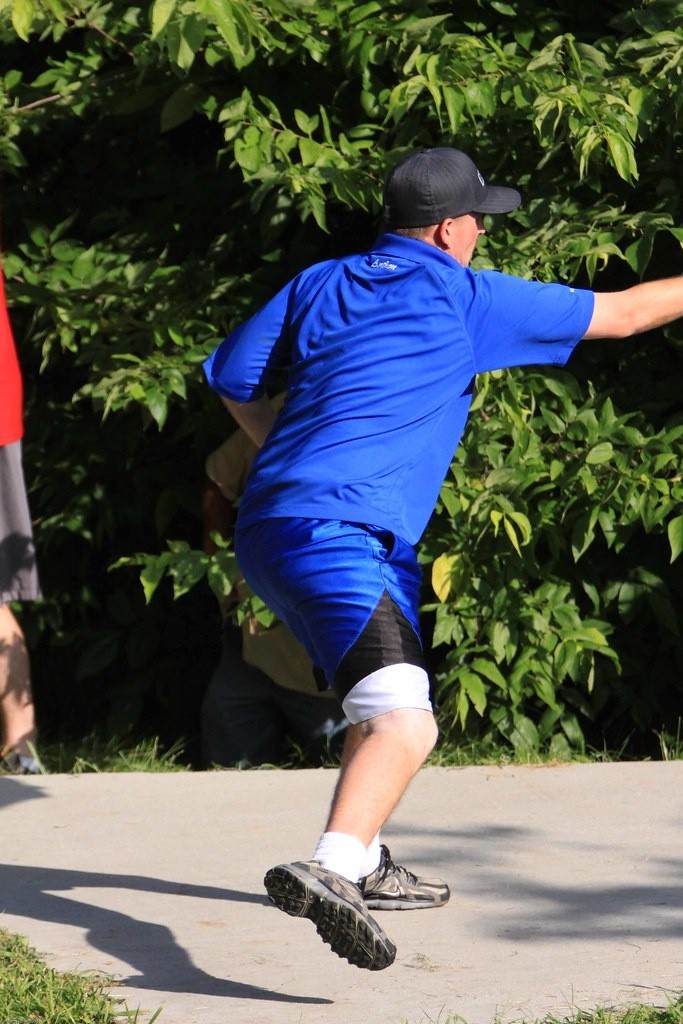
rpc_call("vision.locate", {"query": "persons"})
[0,261,44,773]
[201,146,683,971]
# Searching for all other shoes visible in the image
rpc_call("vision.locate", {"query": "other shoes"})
[0,753,44,774]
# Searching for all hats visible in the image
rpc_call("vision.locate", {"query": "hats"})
[383,147,521,227]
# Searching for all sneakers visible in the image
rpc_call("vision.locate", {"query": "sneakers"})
[265,862,397,972]
[358,845,450,910]
